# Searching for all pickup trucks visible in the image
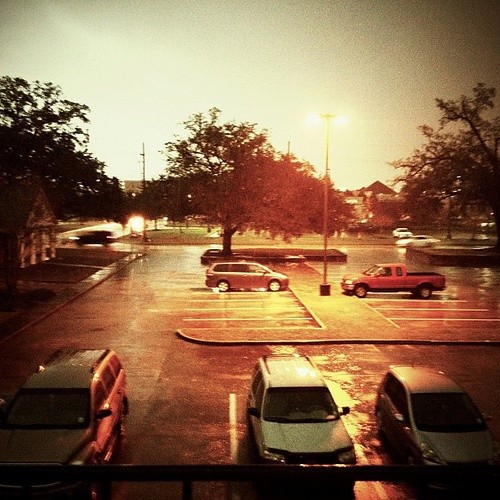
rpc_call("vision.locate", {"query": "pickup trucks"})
[340,263,446,300]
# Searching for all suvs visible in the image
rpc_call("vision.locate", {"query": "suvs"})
[0,347,130,500]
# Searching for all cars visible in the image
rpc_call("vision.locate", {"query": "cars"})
[395,234,442,247]
[205,262,289,292]
[392,228,413,239]
[69,228,118,248]
[246,352,357,492]
[374,364,495,492]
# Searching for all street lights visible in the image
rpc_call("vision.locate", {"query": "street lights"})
[319,112,335,296]
